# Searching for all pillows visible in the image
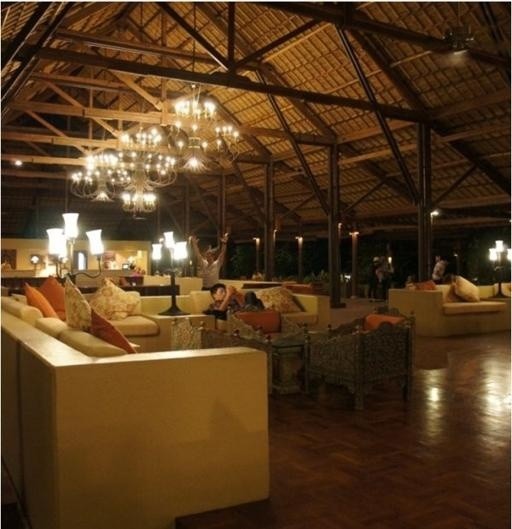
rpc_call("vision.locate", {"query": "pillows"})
[88,276,141,321]
[24,283,60,320]
[65,276,92,332]
[89,307,137,354]
[38,276,67,321]
[455,274,480,302]
[416,279,437,291]
[256,286,304,313]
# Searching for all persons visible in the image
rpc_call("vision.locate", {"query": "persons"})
[207,282,235,320]
[358,255,395,301]
[229,285,264,312]
[189,232,230,291]
[1,257,12,286]
[432,255,449,284]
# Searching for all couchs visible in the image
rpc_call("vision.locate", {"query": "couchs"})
[178,276,256,295]
[177,287,330,377]
[2,295,271,528]
[389,284,510,338]
[494,282,511,295]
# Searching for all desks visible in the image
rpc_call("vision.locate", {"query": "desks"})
[141,312,216,353]
[480,295,510,331]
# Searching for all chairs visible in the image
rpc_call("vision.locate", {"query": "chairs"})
[309,304,417,412]
[285,281,324,295]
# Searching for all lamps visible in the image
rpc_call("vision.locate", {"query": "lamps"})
[116,2,179,221]
[85,228,106,260]
[62,212,81,247]
[170,2,241,171]
[68,49,131,204]
[149,243,164,266]
[46,227,67,265]
